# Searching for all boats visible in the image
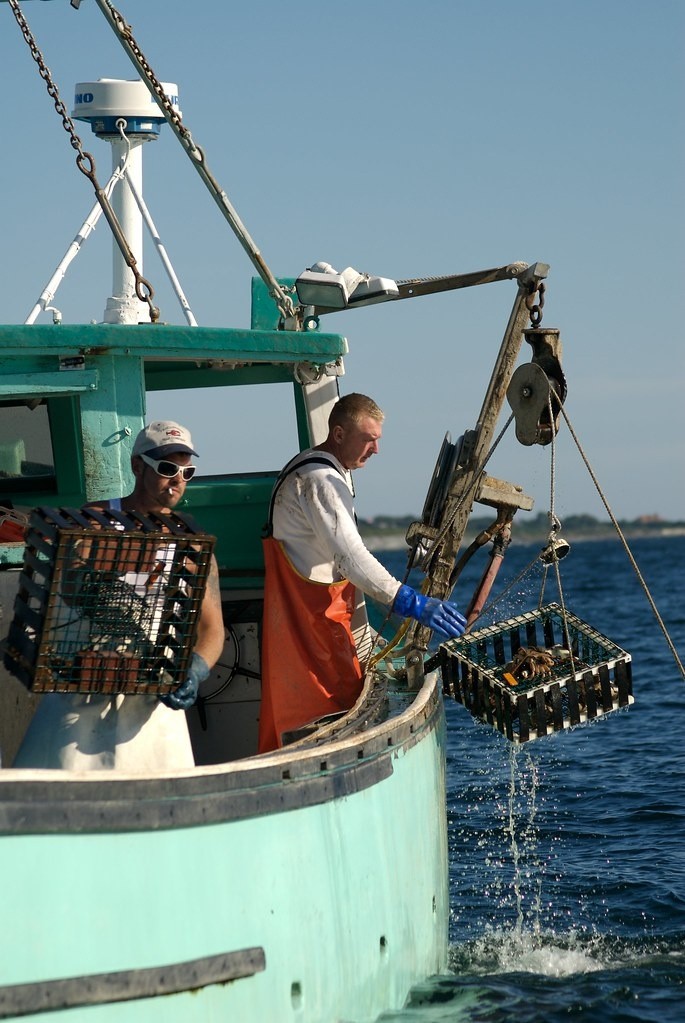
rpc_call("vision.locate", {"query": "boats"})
[0,1,685,1023]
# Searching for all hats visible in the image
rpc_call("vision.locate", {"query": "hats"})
[131,419,199,459]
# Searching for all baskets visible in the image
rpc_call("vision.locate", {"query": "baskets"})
[4,506,213,697]
[438,602,636,745]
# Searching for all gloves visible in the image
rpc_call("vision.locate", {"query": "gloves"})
[0,636,9,661]
[393,584,467,638]
[155,652,211,711]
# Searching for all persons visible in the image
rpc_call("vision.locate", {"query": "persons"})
[11,422,226,771]
[260,393,468,753]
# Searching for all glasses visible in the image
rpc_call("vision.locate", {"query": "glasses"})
[139,453,196,481]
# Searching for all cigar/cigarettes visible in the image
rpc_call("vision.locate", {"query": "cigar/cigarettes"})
[169,488,172,495]
